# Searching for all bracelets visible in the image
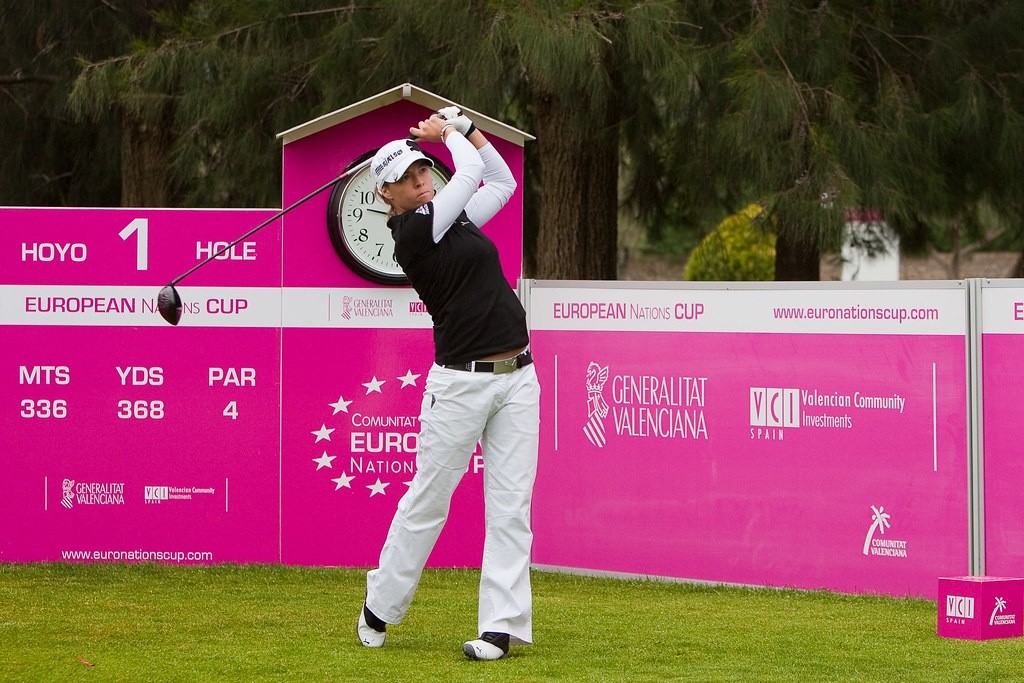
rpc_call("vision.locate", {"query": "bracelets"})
[440,123,454,143]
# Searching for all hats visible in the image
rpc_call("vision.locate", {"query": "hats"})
[370,139,434,192]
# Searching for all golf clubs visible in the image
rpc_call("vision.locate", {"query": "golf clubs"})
[158,113,446,326]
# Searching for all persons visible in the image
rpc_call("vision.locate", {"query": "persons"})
[355,104,542,662]
[838,202,902,284]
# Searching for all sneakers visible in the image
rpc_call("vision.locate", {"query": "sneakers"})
[357,593,387,647]
[463,632,510,661]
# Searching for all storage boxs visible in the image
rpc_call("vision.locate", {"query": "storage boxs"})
[936,575,1024,639]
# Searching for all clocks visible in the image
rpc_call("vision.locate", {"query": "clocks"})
[328,146,455,284]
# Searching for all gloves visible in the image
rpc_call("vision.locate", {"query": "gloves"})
[438,106,476,139]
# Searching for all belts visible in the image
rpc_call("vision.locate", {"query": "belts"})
[437,348,533,375]
